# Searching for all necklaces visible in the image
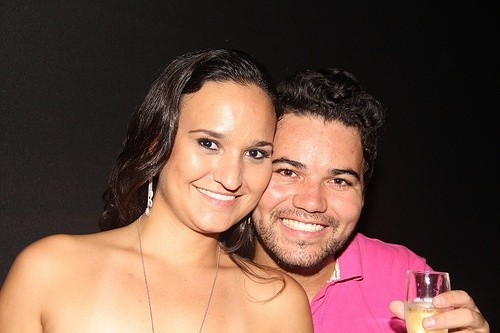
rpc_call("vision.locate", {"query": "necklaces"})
[138,213,222,333]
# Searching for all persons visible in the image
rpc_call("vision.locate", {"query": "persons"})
[1,47,315,333]
[230,68,490,333]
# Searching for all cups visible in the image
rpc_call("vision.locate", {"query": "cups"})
[405,271,451,333]
